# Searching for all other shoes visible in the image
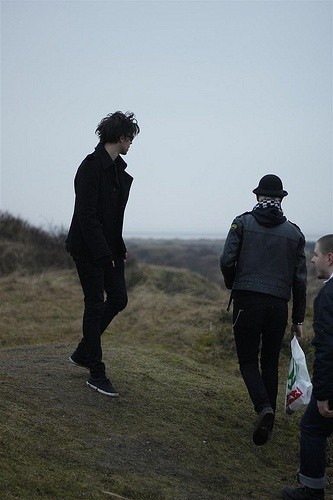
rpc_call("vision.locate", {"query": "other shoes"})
[253,407,275,445]
[282,486,324,500]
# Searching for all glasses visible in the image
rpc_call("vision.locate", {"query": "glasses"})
[126,135,134,142]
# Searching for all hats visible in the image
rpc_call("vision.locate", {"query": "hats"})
[252,174,288,197]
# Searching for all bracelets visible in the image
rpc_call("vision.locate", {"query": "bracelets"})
[294,322,303,326]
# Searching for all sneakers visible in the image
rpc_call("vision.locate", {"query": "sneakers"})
[69,350,91,372]
[86,376,119,397]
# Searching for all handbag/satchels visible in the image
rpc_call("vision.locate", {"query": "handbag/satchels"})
[284,335,313,415]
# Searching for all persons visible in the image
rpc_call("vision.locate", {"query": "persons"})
[220,174,308,447]
[65,110,140,397]
[281,234,333,500]
